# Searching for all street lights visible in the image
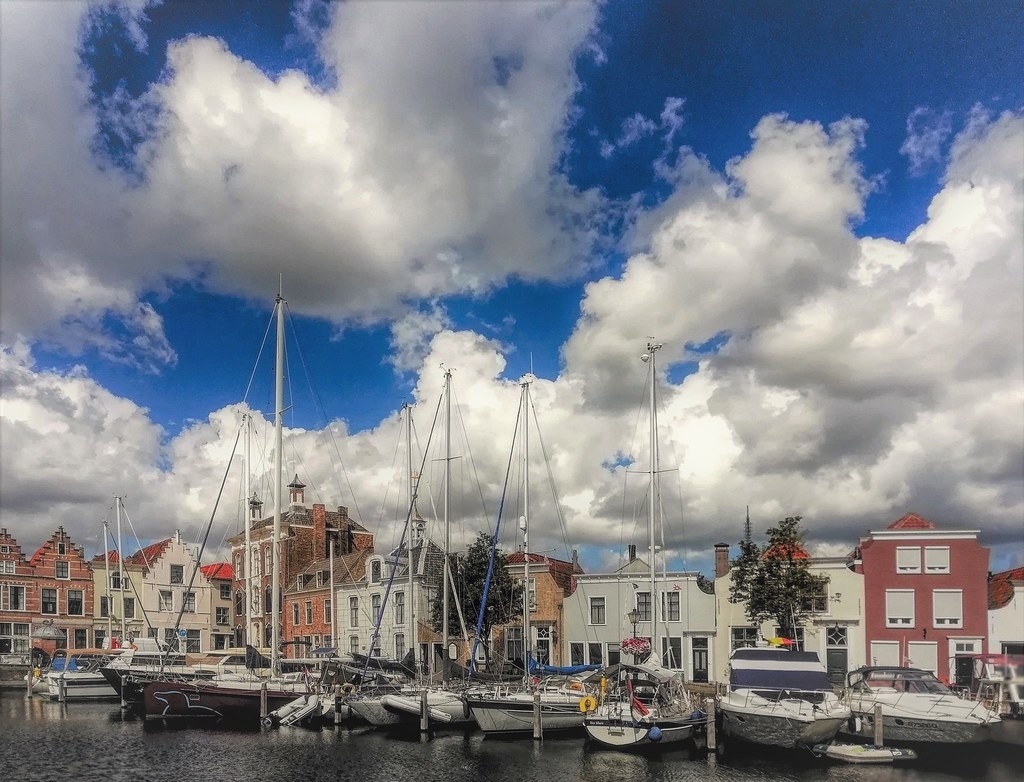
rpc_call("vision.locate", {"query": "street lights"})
[627,607,641,665]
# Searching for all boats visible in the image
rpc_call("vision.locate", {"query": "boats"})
[950,653,1024,748]
[814,742,919,765]
[707,607,852,753]
[837,635,1002,750]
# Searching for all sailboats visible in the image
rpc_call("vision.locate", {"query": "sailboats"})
[25,297,704,750]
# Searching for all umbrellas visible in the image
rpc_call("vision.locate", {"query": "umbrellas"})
[767,638,792,648]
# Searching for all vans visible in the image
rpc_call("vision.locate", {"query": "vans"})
[102,637,175,652]
